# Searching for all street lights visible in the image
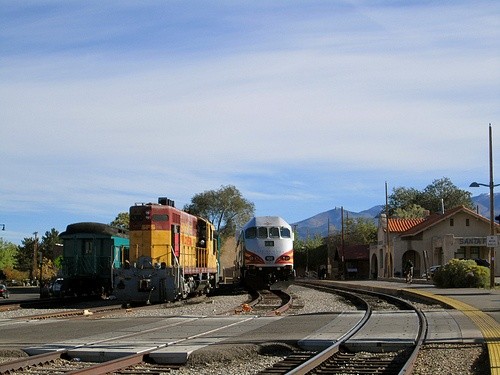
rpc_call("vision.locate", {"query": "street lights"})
[469,123,500,289]
[374,181,403,278]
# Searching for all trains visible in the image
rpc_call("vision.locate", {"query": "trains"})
[236,217,296,280]
[58,222,130,289]
[115,197,220,301]
